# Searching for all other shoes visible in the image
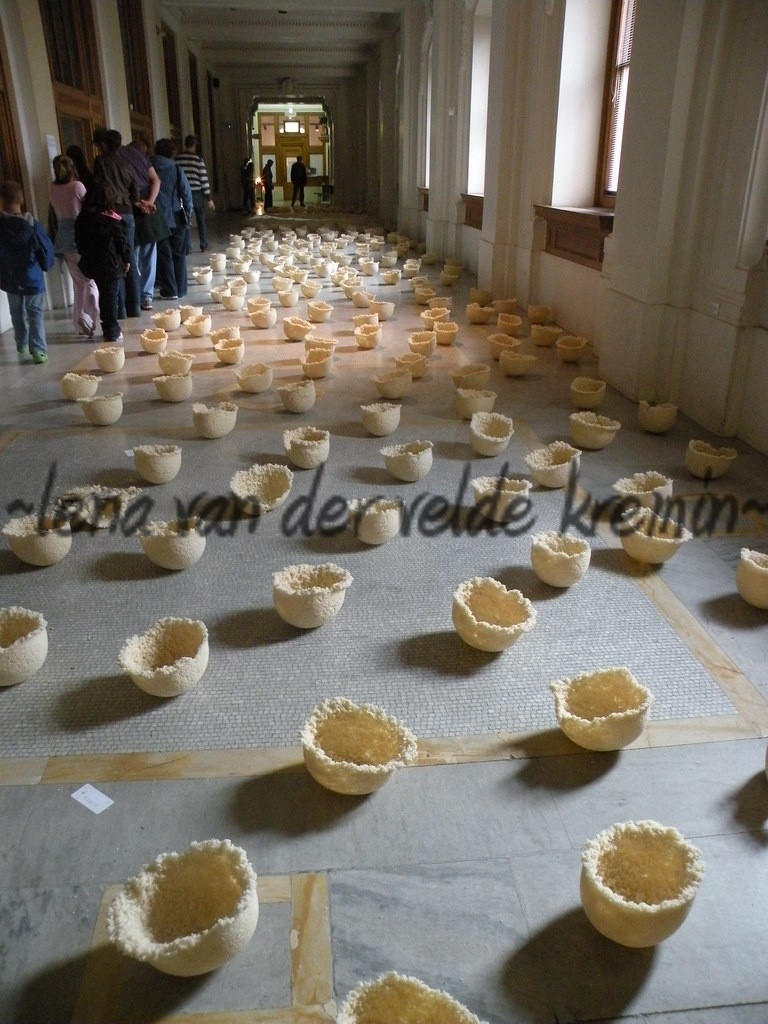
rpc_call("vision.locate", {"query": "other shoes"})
[78,318,93,337]
[17,346,26,353]
[32,352,48,362]
[116,332,123,341]
[141,303,153,309]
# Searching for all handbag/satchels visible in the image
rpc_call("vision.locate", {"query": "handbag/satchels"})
[134,209,173,244]
[175,208,189,227]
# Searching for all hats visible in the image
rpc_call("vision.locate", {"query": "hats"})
[95,128,121,149]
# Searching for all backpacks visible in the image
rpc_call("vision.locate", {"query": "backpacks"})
[74,219,124,284]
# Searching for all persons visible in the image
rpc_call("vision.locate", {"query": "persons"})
[291,156,308,208]
[176,135,215,254]
[262,159,275,209]
[48,129,193,337]
[239,158,258,215]
[1,180,53,363]
[75,179,131,343]
